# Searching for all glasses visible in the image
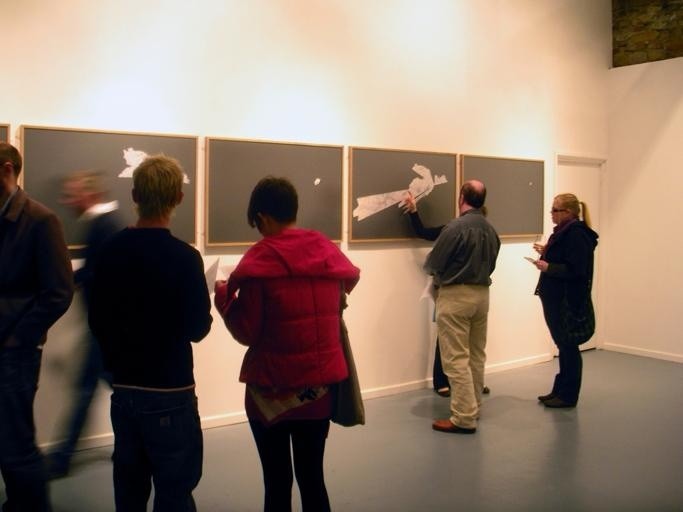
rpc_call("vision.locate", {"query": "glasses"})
[552,207,565,213]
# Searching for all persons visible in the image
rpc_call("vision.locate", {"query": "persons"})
[396,191,490,395]
[534,193,600,408]
[84,155,213,511]
[423,179,501,432]
[213,175,361,511]
[1,142,73,511]
[43,166,130,481]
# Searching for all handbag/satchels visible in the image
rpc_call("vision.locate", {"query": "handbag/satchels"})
[333,316,365,426]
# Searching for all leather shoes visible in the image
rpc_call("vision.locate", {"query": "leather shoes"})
[483,386,489,393]
[438,389,450,397]
[433,420,476,434]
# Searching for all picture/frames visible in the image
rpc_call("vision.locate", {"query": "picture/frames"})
[204,136,345,248]
[461,155,545,238]
[348,146,459,242]
[1,123,10,146]
[20,124,200,250]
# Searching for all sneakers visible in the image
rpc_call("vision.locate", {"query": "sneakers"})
[538,393,576,408]
